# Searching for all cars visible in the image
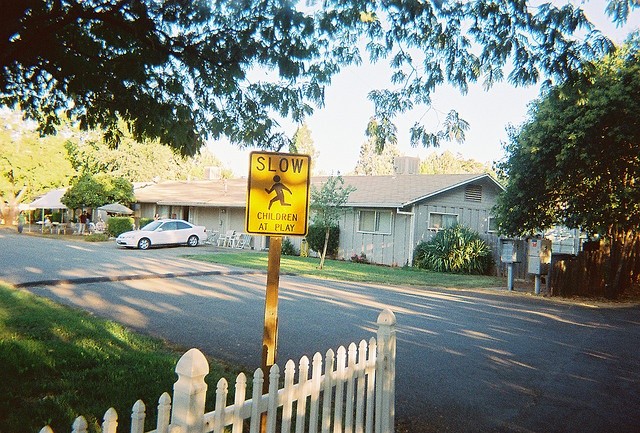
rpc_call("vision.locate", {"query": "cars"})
[115,219,208,250]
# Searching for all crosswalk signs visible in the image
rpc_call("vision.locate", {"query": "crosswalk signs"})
[246,150,311,236]
